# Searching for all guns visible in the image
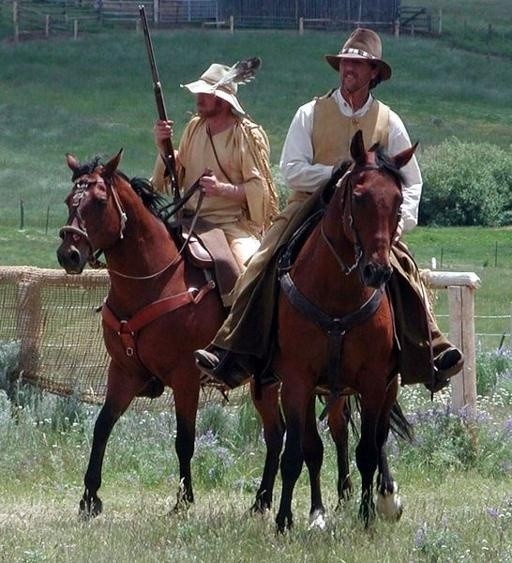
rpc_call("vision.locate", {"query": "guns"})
[136,3,183,221]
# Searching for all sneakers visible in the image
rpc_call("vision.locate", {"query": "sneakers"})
[194,348,226,367]
[433,346,462,371]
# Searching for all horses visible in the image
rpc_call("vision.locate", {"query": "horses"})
[274,128,420,538]
[55,146,354,522]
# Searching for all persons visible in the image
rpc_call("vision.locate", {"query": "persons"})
[192,27,460,390]
[150,63,281,392]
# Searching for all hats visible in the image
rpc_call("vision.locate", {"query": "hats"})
[179,63,245,115]
[324,27,391,81]
